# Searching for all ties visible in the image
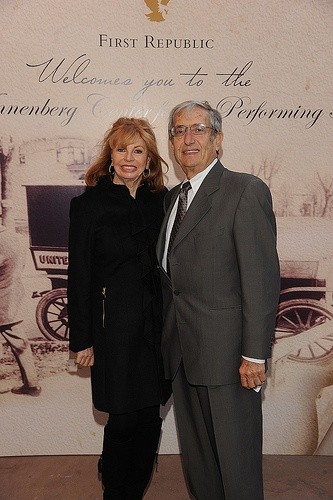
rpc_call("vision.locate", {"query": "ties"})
[166,181,192,279]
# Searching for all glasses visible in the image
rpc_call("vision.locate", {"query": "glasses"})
[168,123,218,137]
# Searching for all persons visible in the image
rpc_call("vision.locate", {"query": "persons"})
[69,117,176,499]
[154,101,282,500]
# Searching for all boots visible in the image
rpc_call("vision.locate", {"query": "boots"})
[101,427,130,500]
[130,419,162,500]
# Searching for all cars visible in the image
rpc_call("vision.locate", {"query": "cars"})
[21,183,333,365]
